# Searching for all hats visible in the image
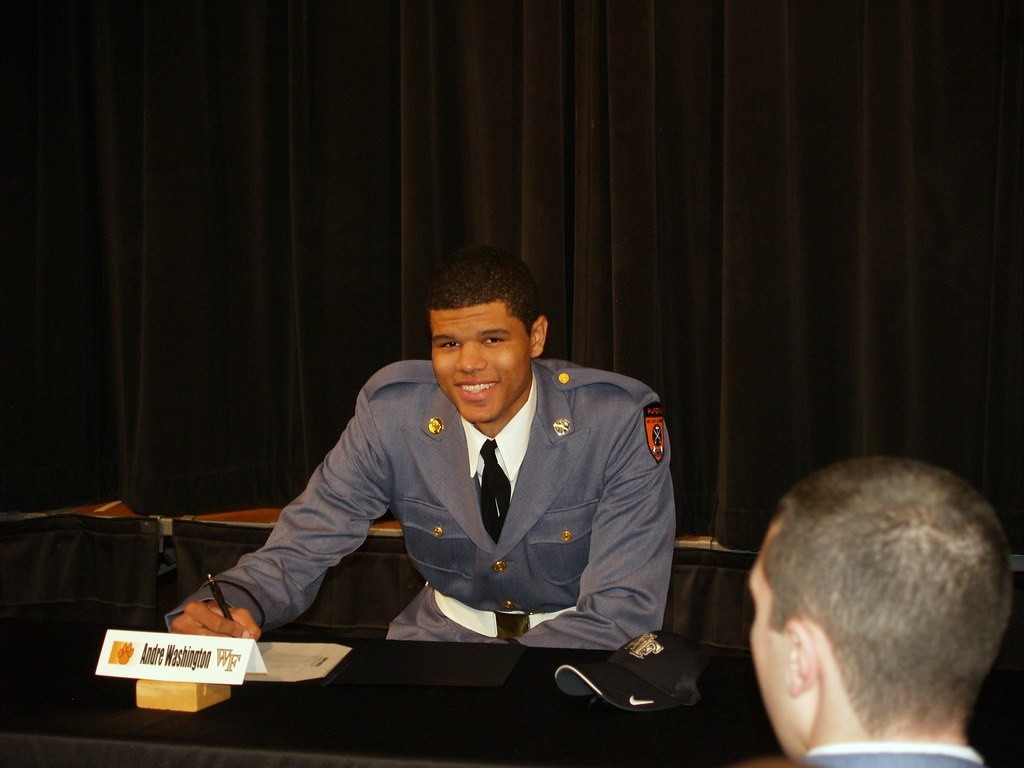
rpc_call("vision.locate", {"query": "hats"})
[554,630,710,709]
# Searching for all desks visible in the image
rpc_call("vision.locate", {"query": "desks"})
[3,589,1024,768]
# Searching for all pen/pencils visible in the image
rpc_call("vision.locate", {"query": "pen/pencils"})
[206,571,235,623]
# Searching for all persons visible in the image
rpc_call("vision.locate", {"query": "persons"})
[163,243,677,650]
[722,453,1015,768]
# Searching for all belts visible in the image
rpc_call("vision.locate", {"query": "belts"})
[435,590,577,639]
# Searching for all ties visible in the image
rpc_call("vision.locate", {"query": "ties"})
[479,439,511,545]
